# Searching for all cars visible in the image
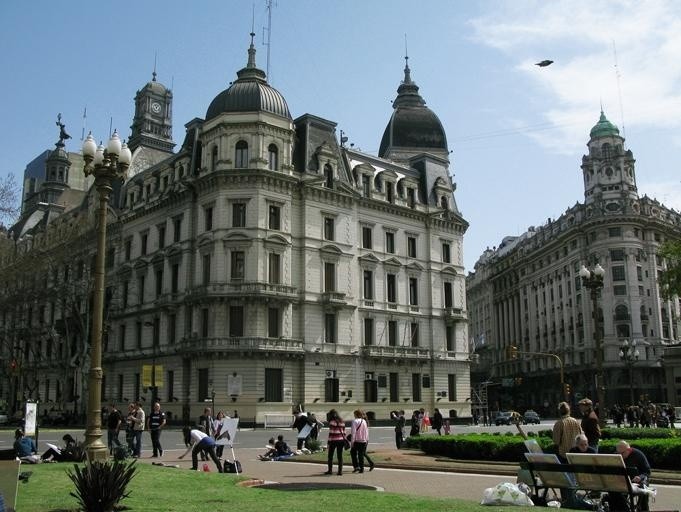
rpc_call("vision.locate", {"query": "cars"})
[0,410,8,425]
[523,409,542,425]
[494,409,524,425]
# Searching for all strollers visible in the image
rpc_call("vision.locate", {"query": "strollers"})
[657,415,670,428]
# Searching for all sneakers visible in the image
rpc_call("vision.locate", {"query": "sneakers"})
[324,463,375,475]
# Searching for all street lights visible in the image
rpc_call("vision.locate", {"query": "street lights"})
[211,388,217,419]
[78,127,133,464]
[579,261,609,431]
[619,338,640,409]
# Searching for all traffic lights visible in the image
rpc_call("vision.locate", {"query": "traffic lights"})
[511,346,518,360]
[565,382,572,395]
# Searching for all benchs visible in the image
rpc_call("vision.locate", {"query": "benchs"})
[522,453,648,512]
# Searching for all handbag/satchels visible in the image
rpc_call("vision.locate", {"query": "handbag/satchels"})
[224,459,243,473]
[343,437,350,451]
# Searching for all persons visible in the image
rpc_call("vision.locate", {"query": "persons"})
[257,407,452,462]
[358,409,376,471]
[100,400,226,474]
[230,409,240,432]
[350,409,370,474]
[325,408,347,475]
[41,434,79,463]
[552,397,677,511]
[13,428,38,465]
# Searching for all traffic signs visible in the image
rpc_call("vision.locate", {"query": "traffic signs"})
[502,378,516,388]
